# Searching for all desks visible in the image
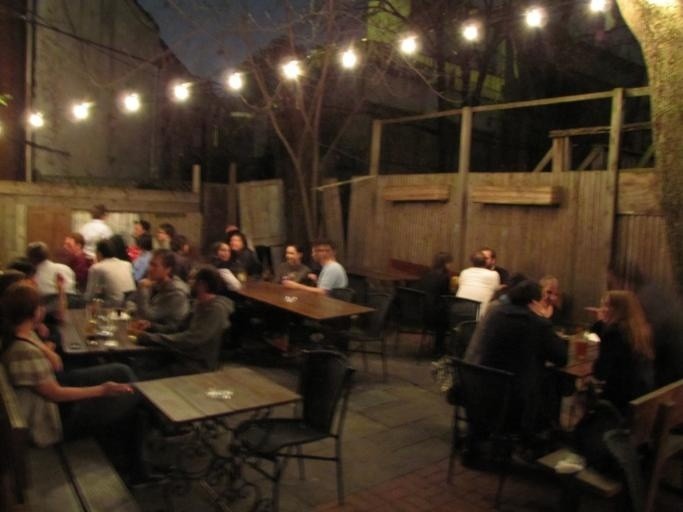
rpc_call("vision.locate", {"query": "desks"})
[240,270,366,351]
[127,362,302,511]
[56,297,161,376]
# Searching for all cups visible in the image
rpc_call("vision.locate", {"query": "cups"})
[83,296,130,346]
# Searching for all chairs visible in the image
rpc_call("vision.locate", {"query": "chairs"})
[230,352,354,509]
[325,269,481,383]
[448,316,683,512]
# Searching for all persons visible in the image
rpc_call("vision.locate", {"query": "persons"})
[278,239,348,296]
[555,290,657,423]
[1,203,193,336]
[0,282,195,487]
[464,280,568,456]
[126,263,232,377]
[211,224,263,288]
[412,245,574,361]
